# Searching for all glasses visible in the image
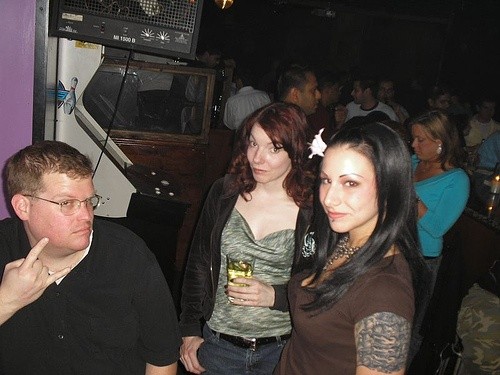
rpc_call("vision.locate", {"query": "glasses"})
[22,193,102,216]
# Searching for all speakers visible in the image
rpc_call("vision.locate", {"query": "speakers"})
[48,0,204,60]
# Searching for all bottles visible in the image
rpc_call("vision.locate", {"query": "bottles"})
[211,97,222,128]
[486,173,500,210]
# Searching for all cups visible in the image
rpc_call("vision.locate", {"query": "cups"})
[227,253,256,304]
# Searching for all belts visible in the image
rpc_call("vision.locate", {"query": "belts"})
[211,330,291,348]
[422,256,437,261]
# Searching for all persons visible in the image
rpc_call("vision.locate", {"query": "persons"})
[179,102,317,375]
[272,116,426,375]
[185,47,500,168]
[0,140,184,375]
[408,109,471,360]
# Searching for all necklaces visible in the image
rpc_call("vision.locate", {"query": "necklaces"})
[322,233,361,270]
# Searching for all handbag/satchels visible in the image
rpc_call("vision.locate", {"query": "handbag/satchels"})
[414,334,464,375]
